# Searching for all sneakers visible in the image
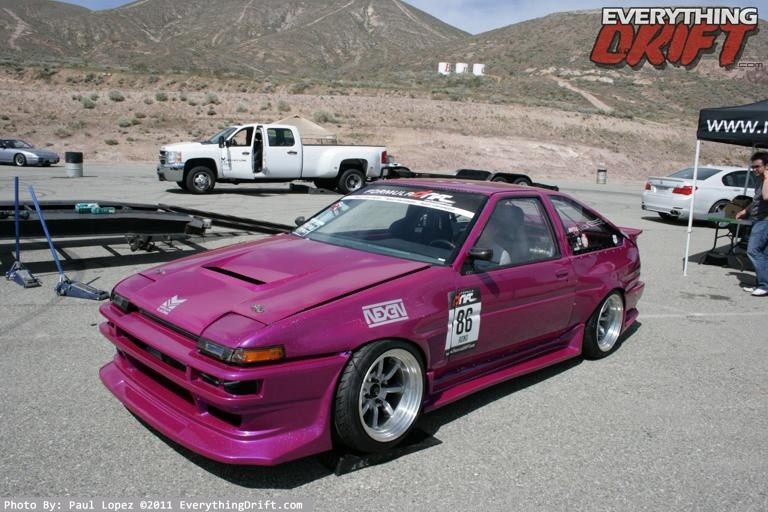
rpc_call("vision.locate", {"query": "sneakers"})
[752,288,768,295]
[743,287,755,292]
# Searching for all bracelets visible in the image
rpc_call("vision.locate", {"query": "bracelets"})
[764,176,767,180]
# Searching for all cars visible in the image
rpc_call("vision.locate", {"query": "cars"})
[95,169,645,468]
[0,137,59,168]
[641,164,757,229]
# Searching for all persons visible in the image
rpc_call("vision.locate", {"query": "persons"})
[736,152,767,296]
[473,217,511,266]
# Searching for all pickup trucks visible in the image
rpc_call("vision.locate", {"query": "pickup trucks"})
[156,123,389,197]
